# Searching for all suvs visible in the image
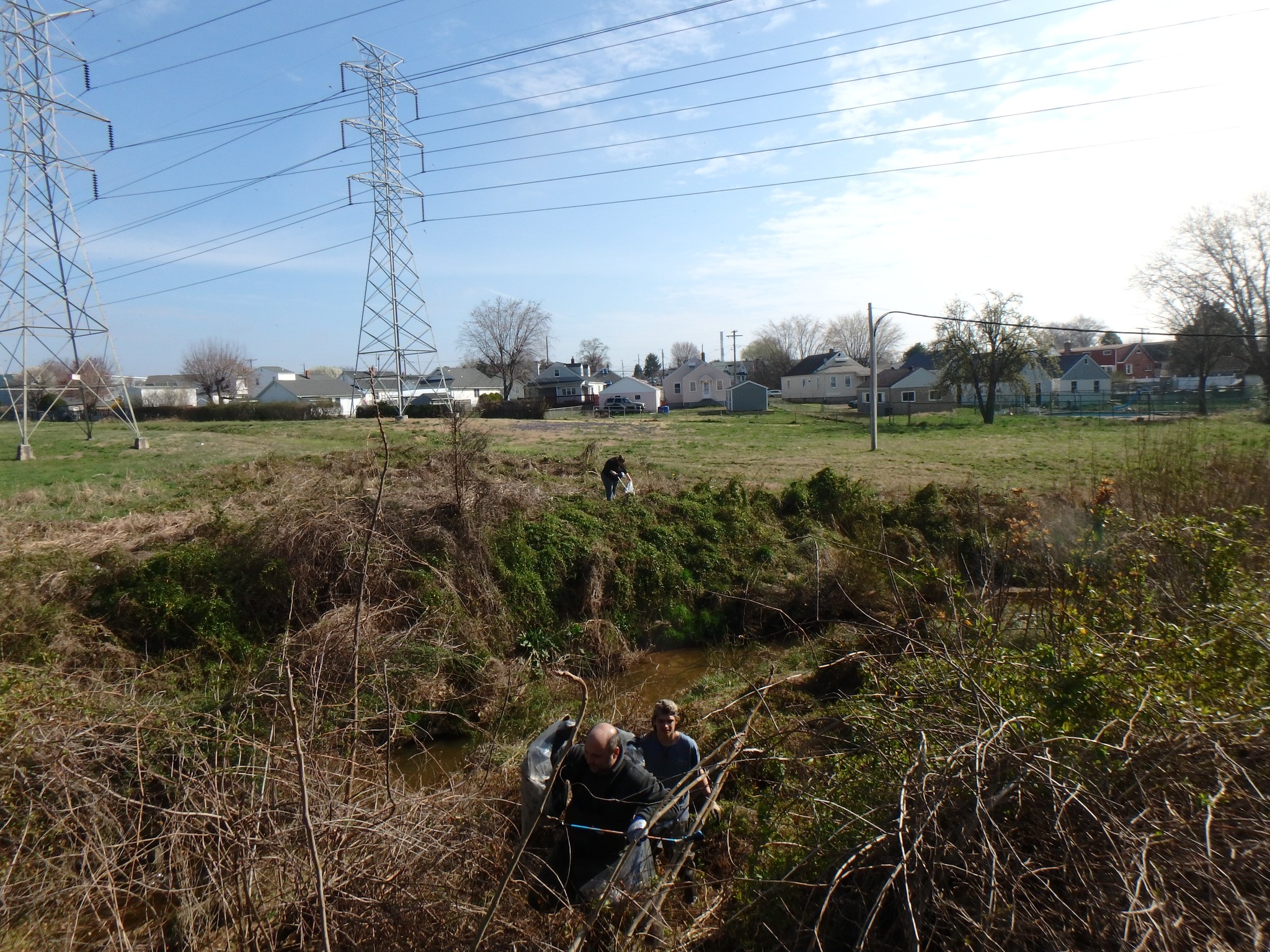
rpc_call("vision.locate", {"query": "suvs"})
[603,396,646,415]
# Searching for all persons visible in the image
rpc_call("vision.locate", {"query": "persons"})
[641,699,723,904]
[544,722,680,950]
[601,455,631,501]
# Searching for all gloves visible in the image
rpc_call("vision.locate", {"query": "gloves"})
[610,470,616,475]
[555,716,574,740]
[705,798,722,824]
[626,817,648,846]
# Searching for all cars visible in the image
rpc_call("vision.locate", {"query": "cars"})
[848,400,857,409]
[767,389,782,398]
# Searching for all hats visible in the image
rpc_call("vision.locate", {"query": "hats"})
[654,699,679,718]
